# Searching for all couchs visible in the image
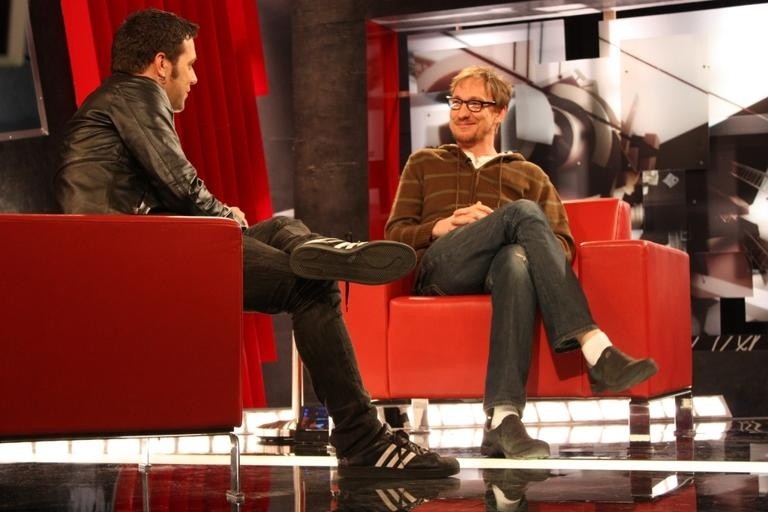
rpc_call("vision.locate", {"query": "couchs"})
[336,198,692,446]
[1,214,244,503]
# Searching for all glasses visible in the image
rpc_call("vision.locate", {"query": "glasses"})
[445,95,496,112]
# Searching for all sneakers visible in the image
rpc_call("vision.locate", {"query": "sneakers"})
[337,479,459,512]
[290,231,416,285]
[338,422,460,480]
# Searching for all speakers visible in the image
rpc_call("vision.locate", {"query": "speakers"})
[564,19,599,61]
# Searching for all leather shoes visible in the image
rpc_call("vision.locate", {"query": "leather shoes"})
[483,469,551,501]
[480,414,550,458]
[586,345,658,395]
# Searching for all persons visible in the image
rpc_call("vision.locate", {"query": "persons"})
[338,478,461,512]
[478,469,552,512]
[51,6,459,478]
[382,65,661,459]
